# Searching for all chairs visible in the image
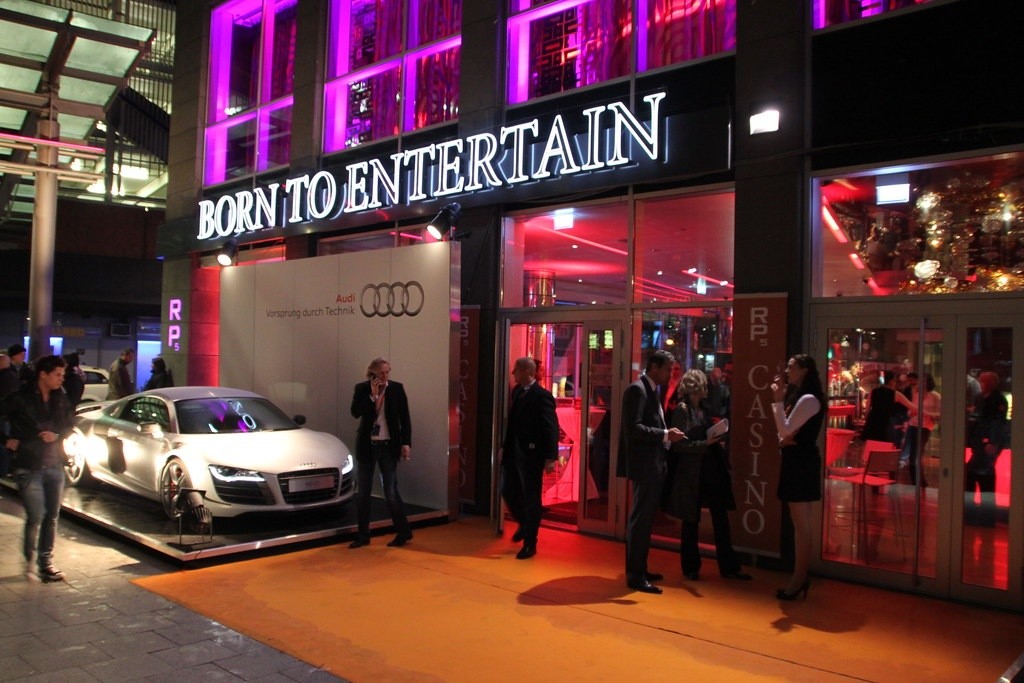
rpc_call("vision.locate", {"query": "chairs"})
[829,448,910,563]
[825,428,856,468]
[824,437,898,549]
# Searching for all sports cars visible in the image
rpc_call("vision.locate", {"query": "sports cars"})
[58,385,357,522]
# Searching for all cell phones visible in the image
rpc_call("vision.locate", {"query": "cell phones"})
[368,372,382,389]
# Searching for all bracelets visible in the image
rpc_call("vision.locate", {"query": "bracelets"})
[55,432,60,441]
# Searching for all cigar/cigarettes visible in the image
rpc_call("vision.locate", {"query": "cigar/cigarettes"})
[401,456,411,460]
[683,435,688,439]
[61,385,67,394]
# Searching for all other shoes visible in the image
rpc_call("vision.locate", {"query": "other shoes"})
[350,537,370,548]
[725,569,754,581]
[387,531,413,546]
[686,570,699,580]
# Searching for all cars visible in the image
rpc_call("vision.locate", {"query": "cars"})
[73,364,111,403]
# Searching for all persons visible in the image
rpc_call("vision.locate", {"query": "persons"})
[0,356,75,583]
[667,365,755,580]
[350,358,414,548]
[142,357,172,391]
[659,358,686,411]
[860,373,938,476]
[890,371,919,484]
[699,366,731,418]
[770,353,828,602]
[0,344,34,477]
[616,348,689,595]
[105,347,136,399]
[59,351,86,409]
[497,357,559,560]
[908,372,942,487]
[966,371,1007,527]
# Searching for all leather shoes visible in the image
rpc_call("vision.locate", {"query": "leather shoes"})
[648,574,663,580]
[638,582,662,593]
[516,544,536,559]
[513,525,523,542]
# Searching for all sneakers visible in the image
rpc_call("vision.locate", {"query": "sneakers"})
[38,566,66,580]
[25,560,36,581]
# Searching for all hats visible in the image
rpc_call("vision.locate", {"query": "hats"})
[8,344,26,356]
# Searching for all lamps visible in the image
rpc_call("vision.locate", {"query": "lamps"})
[217,237,241,267]
[166,487,225,553]
[425,202,467,242]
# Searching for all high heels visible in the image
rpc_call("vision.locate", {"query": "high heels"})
[777,579,809,600]
